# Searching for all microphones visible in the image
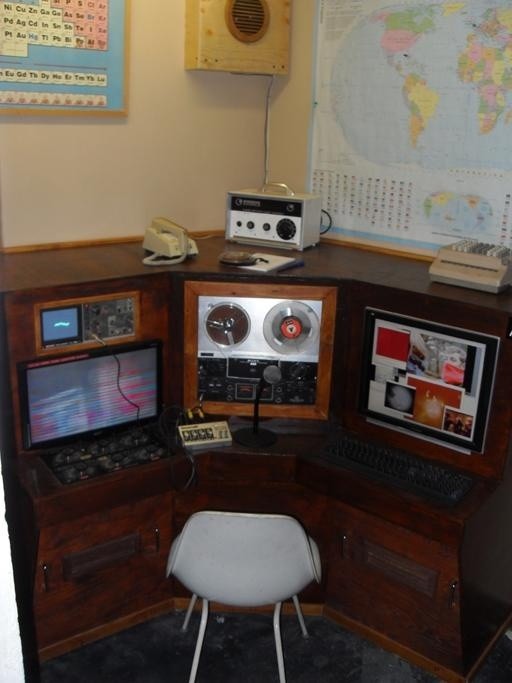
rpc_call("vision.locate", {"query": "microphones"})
[235,364,285,447]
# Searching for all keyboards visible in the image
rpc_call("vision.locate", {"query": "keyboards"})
[316,431,481,509]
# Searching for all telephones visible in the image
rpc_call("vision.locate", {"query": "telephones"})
[142,217,200,258]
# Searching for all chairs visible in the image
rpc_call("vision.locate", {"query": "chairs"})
[165,511,323,683]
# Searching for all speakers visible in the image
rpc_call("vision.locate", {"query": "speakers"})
[183,0,293,77]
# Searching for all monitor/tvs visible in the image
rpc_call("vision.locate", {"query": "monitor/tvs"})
[16,338,164,451]
[354,304,501,456]
